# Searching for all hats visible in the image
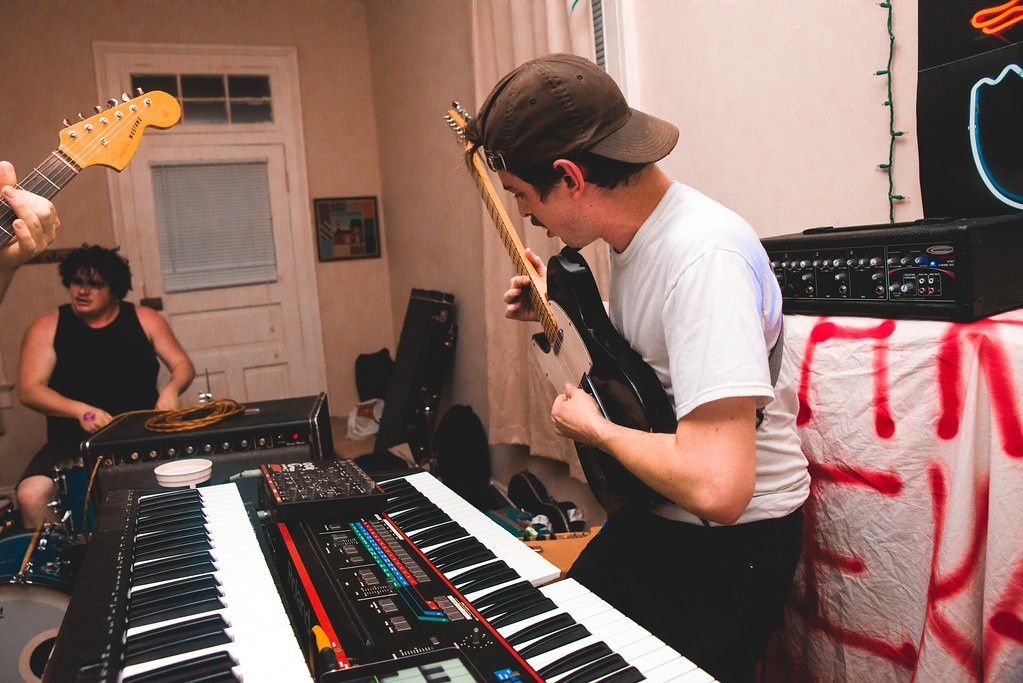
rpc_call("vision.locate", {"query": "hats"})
[476,52,680,172]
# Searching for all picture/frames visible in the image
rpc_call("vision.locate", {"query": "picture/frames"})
[313,195,382,263]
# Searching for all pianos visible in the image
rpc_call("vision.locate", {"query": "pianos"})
[38,469,723,683]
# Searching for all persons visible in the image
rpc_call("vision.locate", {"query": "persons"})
[16,246,197,530]
[1,160,61,308]
[464,53,812,682]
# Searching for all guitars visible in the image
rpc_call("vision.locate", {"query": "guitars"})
[0,85,183,253]
[443,100,677,525]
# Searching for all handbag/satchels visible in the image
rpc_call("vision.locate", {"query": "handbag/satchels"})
[345,398,385,440]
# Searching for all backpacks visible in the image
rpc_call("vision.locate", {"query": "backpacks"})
[430,404,491,507]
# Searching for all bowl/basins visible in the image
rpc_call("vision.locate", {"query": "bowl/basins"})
[153,458,213,487]
